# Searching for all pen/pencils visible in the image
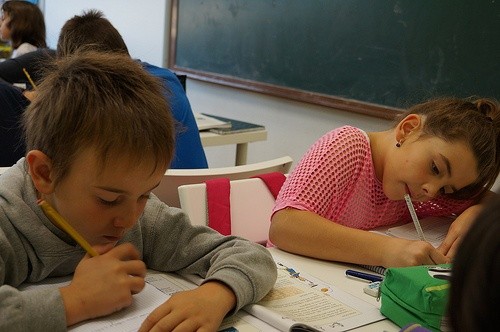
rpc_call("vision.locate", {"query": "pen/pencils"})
[345,269,384,283]
[37,199,101,257]
[22,68,37,88]
[404,193,426,241]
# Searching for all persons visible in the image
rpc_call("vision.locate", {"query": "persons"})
[449,189,500,332]
[269,96,500,267]
[0,0,56,167]
[56,15,209,169]
[0,53,278,332]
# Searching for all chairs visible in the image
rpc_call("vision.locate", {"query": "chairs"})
[153,156,292,245]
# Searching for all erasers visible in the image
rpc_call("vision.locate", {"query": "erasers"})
[363,281,382,298]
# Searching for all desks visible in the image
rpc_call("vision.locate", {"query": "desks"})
[194,113,268,167]
[49,213,464,332]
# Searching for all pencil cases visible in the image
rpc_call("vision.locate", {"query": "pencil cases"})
[379,262,455,332]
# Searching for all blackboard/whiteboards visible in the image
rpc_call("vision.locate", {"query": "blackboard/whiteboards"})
[162,0,500,122]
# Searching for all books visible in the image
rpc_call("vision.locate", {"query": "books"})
[193,112,265,135]
[20,215,455,332]
